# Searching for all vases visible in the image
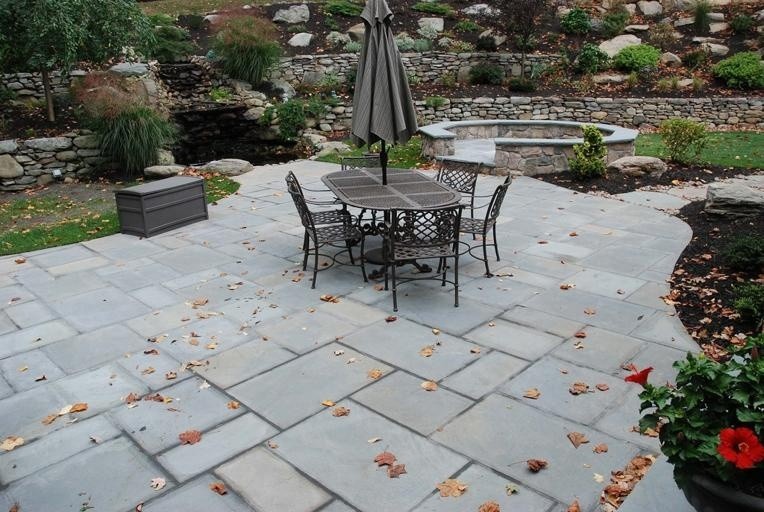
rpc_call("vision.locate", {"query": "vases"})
[686,472,763,512]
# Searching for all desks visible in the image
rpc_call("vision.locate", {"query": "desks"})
[320,166,462,289]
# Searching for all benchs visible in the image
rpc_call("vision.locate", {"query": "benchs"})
[418,119,639,175]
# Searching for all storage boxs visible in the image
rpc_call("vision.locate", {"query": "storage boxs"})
[114,175,209,239]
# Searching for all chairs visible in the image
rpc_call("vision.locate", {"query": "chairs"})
[383,205,464,316]
[285,170,355,268]
[436,174,513,275]
[287,182,371,289]
[338,153,387,242]
[433,157,485,242]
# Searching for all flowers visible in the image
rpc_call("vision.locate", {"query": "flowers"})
[619,334,763,499]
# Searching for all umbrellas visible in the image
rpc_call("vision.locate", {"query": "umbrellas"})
[349,1,418,267]
